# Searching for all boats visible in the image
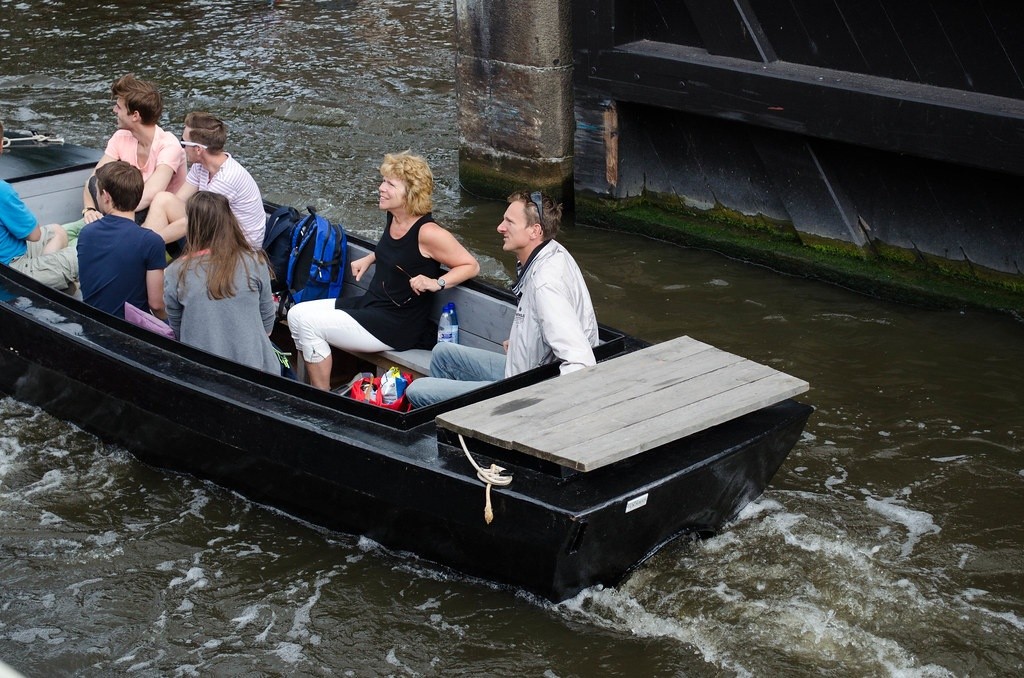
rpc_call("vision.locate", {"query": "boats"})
[0,129,815,606]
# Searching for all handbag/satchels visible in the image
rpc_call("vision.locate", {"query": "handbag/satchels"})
[350,372,413,413]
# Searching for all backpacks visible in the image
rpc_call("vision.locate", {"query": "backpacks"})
[287,207,347,305]
[263,207,301,291]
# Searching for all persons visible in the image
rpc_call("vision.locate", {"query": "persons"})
[162,190,299,381]
[287,150,481,392]
[0,121,79,291]
[405,186,600,409]
[140,111,267,260]
[82,73,188,226]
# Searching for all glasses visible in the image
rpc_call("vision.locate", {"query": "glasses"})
[181,138,207,149]
[531,191,543,222]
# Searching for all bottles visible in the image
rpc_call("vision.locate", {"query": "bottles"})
[448,303,458,343]
[437,307,452,344]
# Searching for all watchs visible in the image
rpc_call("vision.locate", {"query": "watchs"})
[82,207,96,217]
[437,278,446,289]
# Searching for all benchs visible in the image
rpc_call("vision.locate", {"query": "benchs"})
[263,212,608,381]
